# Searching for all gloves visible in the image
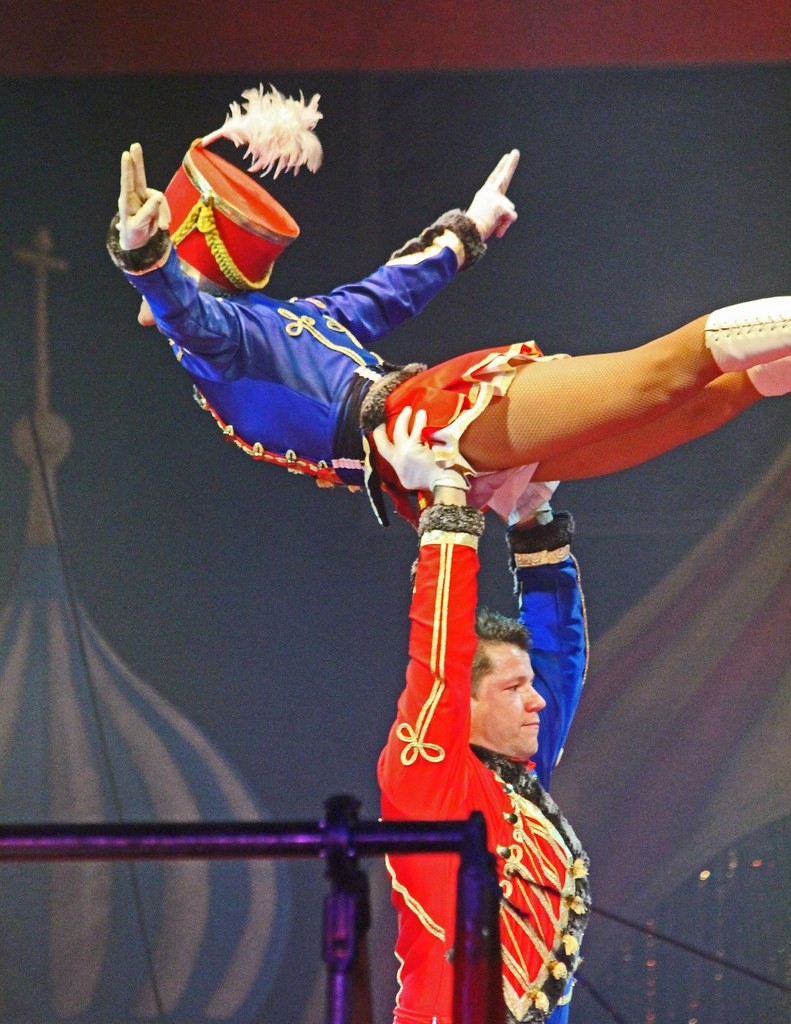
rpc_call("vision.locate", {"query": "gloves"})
[508,480,560,525]
[372,406,472,494]
[115,142,170,250]
[466,149,520,243]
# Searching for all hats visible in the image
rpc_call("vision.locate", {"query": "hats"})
[162,81,323,294]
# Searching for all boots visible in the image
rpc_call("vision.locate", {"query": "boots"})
[746,356,791,397]
[704,296,791,372]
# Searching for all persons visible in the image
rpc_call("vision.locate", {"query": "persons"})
[375,407,591,1024]
[106,83,791,532]
